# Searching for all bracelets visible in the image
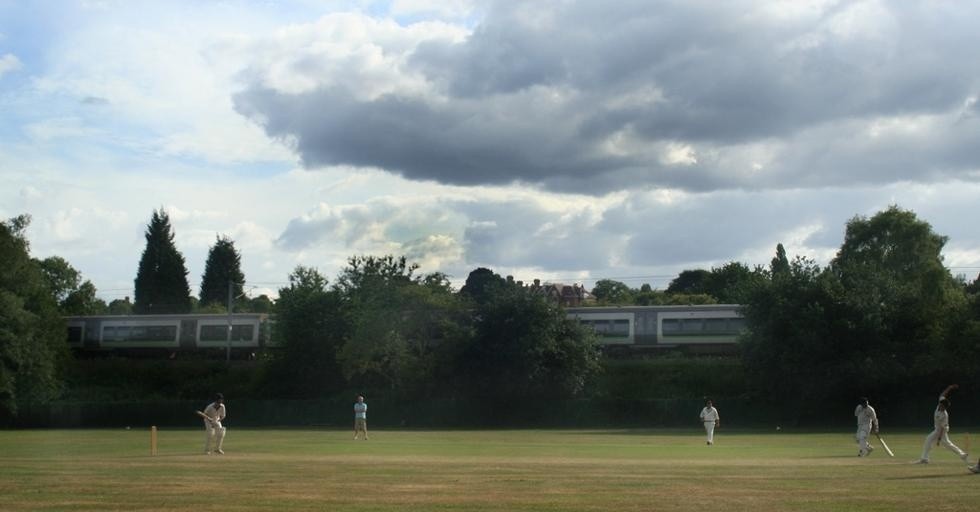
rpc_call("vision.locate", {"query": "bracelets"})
[716,423,719,424]
[938,437,941,440]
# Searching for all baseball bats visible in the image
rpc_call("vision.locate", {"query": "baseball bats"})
[196,410,213,422]
[877,433,894,457]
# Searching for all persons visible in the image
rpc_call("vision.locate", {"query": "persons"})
[699,397,721,445]
[854,397,880,458]
[916,385,968,464]
[354,397,372,441]
[203,393,226,455]
[967,456,980,474]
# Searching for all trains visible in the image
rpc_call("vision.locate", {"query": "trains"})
[58,302,884,368]
